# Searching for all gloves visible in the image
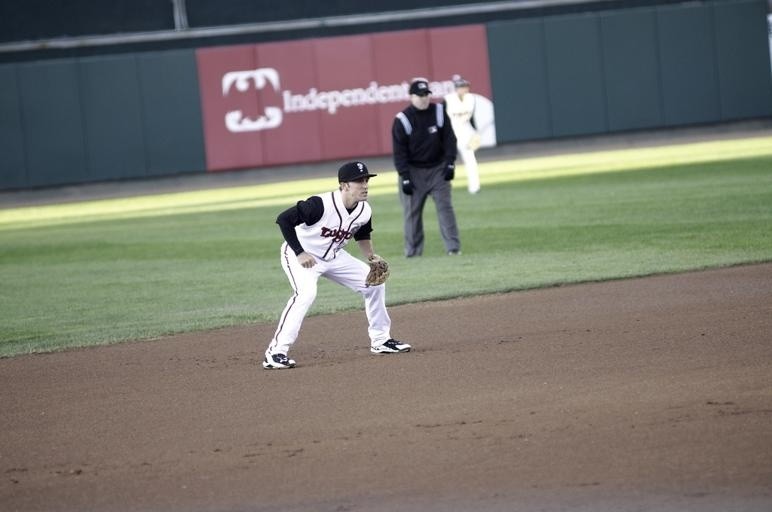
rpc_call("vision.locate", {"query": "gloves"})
[401,174,415,196]
[441,161,454,181]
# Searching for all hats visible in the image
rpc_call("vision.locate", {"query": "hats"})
[338,162,378,183]
[454,79,468,87]
[410,81,432,96]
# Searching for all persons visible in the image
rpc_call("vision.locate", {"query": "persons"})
[442,80,483,194]
[391,81,461,257]
[262,162,411,369]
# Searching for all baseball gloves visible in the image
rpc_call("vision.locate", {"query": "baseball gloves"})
[365,255,390,286]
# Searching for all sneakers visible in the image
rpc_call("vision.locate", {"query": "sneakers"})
[371,339,412,355]
[262,354,296,370]
